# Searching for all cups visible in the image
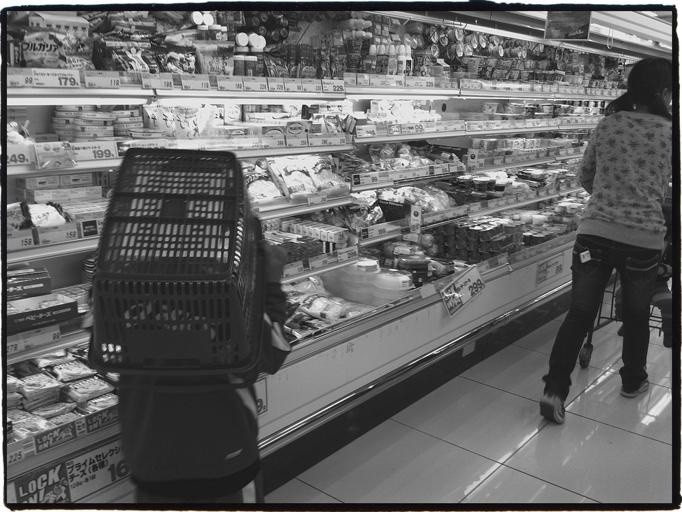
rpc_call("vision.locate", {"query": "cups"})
[404,23,584,73]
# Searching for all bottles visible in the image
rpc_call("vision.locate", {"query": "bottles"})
[368,45,413,76]
[185,12,267,76]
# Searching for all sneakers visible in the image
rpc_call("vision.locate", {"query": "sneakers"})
[540,393,565,424]
[620,380,649,398]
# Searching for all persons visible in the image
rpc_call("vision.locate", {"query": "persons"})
[616,201,674,347]
[539,57,674,425]
[88,215,291,504]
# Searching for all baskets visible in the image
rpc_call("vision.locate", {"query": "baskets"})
[86,150,264,375]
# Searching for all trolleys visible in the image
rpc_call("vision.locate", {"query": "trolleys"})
[581,261,670,369]
